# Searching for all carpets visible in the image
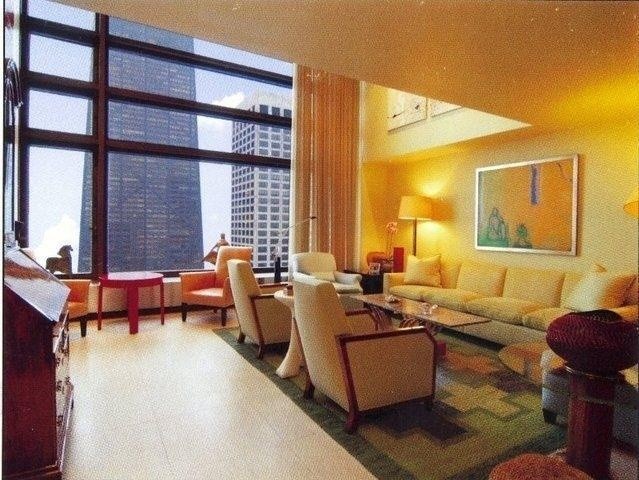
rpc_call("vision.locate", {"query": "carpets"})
[213,326,568,480]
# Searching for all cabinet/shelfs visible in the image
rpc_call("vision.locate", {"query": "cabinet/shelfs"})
[4,58,75,480]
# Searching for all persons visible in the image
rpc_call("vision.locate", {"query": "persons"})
[512,221,533,248]
[486,205,506,243]
[201,234,230,265]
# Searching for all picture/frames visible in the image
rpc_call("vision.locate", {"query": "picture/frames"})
[473,154,579,257]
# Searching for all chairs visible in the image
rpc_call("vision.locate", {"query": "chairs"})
[59,279,90,337]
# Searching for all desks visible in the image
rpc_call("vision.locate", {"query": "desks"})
[97,271,165,335]
[343,268,383,292]
[540,361,638,463]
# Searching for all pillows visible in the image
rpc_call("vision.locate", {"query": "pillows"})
[563,264,634,311]
[403,255,442,287]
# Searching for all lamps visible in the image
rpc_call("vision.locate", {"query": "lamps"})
[274,216,317,282]
[398,195,435,256]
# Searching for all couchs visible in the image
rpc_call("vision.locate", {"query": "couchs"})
[229,260,291,359]
[291,253,364,309]
[383,253,639,349]
[180,245,253,327]
[290,277,435,433]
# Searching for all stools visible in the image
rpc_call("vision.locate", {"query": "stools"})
[489,454,594,480]
